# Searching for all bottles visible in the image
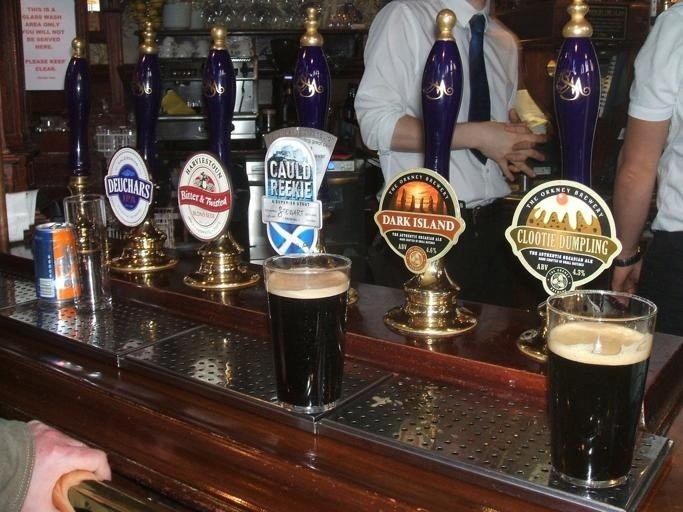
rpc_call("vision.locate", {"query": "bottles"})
[31,222,81,304]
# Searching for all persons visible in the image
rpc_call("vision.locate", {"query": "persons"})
[353,0,551,306]
[0,417,113,512]
[610,1,683,336]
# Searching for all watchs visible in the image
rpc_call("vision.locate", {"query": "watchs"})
[613,245,642,267]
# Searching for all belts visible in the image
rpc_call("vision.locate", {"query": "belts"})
[458,199,504,221]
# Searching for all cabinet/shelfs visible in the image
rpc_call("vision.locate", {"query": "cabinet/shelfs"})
[0,0,683,206]
[4,249,682,511]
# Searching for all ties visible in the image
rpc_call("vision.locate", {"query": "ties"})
[468,13,490,165]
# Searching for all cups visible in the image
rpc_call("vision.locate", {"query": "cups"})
[263,253,351,411]
[547,291,657,488]
[65,194,112,310]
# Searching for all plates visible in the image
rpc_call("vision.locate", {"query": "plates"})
[322,372,673,512]
[4,297,210,370]
[125,324,395,435]
[1,272,84,312]
